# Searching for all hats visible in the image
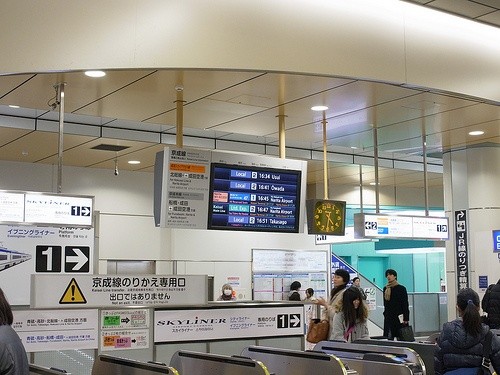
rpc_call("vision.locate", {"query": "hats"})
[332,269,349,277]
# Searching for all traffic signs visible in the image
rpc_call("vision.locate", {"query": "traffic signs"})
[362,214,449,243]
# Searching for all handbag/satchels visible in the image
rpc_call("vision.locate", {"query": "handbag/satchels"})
[306,319,330,343]
[398,322,415,341]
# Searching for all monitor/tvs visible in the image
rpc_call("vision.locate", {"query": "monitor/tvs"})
[206,162,301,233]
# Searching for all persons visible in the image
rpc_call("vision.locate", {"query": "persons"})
[433,288,500,375]
[383,269,410,340]
[316,269,350,340]
[0,288,28,375]
[330,287,370,342]
[481,279,500,329]
[351,277,367,300]
[288,281,301,301]
[304,288,315,301]
[217,284,236,301]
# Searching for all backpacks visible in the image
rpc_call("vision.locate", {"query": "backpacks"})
[436,326,495,375]
